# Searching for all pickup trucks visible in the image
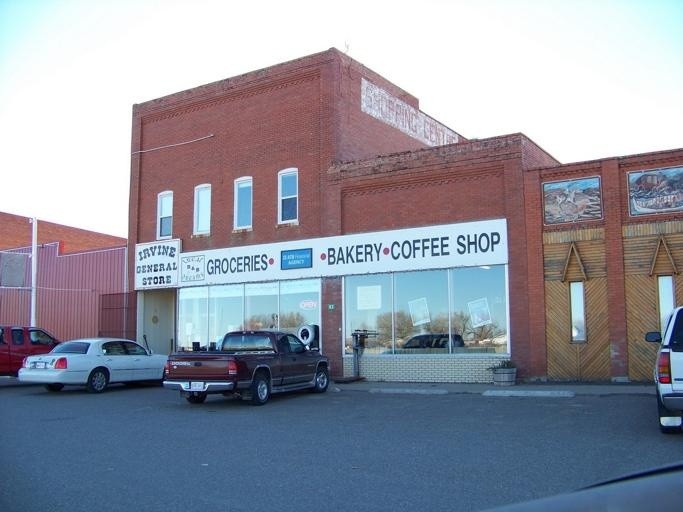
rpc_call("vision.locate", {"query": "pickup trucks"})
[0,324,69,378]
[159,329,333,406]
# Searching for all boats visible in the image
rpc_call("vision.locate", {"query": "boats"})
[629,187,683,215]
[574,190,602,220]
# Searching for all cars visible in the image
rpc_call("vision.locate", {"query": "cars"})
[17,336,170,394]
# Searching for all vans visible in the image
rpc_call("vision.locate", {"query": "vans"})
[643,304,683,436]
[401,331,464,348]
